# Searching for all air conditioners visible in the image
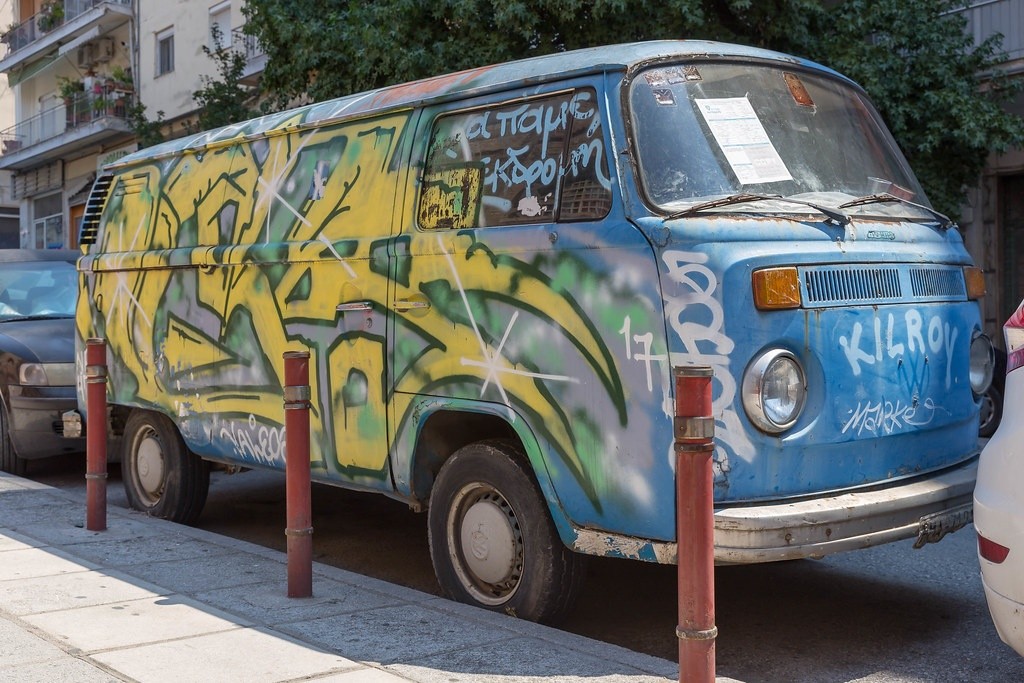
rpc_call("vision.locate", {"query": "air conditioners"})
[77,45,95,69]
[93,39,112,62]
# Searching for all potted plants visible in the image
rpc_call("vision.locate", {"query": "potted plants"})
[69,103,80,121]
[77,102,91,122]
[94,81,102,93]
[113,66,126,89]
[52,75,74,105]
[103,75,114,87]
[92,98,116,116]
[122,72,136,89]
[14,21,25,37]
[71,80,85,100]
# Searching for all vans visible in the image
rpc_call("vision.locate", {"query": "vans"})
[75,39,996,627]
[0,249,125,479]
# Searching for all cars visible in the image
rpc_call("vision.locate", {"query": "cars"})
[979,346,1007,437]
[973,299,1024,659]
